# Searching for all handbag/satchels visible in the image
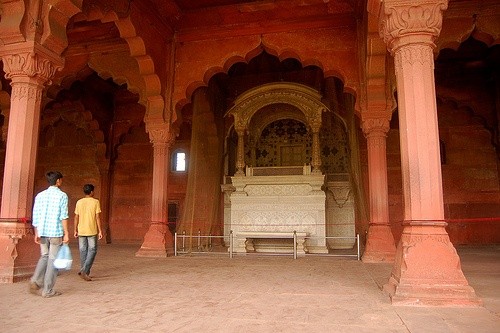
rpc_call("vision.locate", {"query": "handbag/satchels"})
[53,243,73,269]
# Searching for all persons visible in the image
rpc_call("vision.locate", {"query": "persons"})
[73,184,103,281]
[27,170,69,298]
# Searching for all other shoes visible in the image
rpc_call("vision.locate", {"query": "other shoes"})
[42,290,62,298]
[29,281,40,294]
[78,272,92,281]
